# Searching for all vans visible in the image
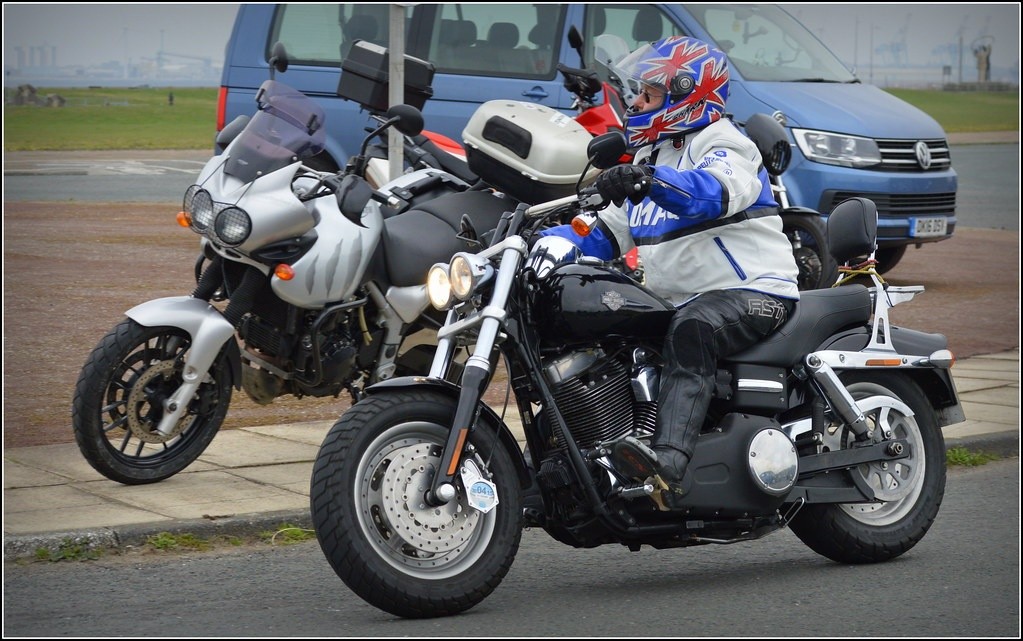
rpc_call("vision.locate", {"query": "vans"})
[214,3,959,291]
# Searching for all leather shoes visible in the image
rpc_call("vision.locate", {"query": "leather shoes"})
[614,436,688,486]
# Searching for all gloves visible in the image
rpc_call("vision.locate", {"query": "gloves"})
[519,230,543,255]
[596,163,650,205]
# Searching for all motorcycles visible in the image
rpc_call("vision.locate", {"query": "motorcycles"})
[71,24,838,485]
[310,131,968,619]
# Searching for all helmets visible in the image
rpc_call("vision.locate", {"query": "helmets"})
[622,35,728,148]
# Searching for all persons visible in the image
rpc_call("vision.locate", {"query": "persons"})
[483,37,802,499]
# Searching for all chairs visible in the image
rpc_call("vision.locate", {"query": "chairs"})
[484,23,532,75]
[633,9,663,50]
[440,17,479,73]
[339,14,381,62]
[594,6,606,36]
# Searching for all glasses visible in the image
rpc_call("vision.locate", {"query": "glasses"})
[640,89,661,103]
